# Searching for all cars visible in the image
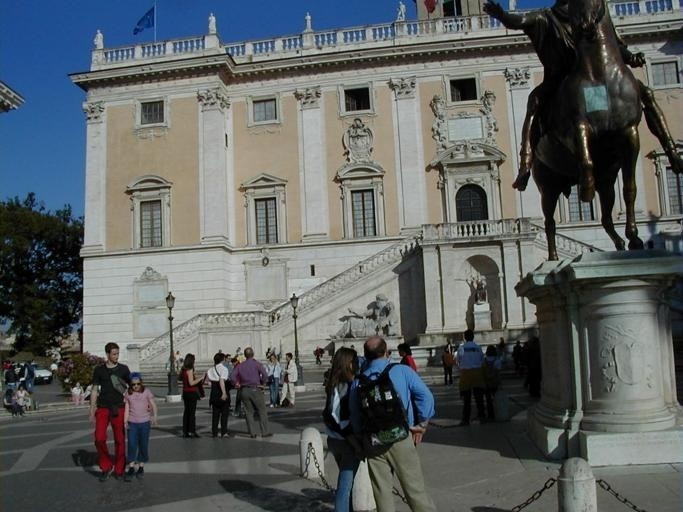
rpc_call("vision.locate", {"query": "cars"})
[3,362,53,385]
[492,341,526,376]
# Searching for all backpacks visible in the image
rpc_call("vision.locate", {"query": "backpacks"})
[354,363,409,449]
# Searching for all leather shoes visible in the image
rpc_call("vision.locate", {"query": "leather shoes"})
[251,434,257,438]
[262,434,273,438]
[183,432,201,438]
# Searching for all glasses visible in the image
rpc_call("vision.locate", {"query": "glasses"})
[131,383,142,386]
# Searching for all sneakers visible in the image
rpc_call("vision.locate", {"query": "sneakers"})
[99,465,145,481]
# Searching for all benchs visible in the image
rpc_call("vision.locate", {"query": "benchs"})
[52,386,92,404]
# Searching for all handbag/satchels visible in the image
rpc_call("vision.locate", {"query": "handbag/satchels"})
[219,380,233,394]
[267,375,275,386]
[282,398,294,408]
[110,374,127,395]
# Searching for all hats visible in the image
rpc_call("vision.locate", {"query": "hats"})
[129,372,141,380]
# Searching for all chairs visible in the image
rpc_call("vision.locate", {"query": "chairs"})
[1,390,34,418]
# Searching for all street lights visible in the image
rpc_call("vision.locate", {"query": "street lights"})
[165,291,177,377]
[289,293,301,368]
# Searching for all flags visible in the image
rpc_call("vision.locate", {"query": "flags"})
[133,6,154,35]
[423,0,436,13]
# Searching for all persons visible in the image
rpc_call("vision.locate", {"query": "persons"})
[208,12,216,33]
[305,12,312,30]
[482,0,683,192]
[93,29,103,49]
[395,1,406,22]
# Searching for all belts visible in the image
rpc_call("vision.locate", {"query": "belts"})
[241,385,258,388]
